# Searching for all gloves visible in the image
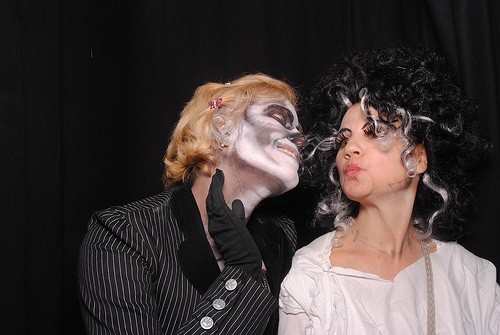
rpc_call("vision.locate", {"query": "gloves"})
[206,168,263,279]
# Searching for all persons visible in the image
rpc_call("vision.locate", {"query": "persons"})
[278,48,499,334]
[78,73,309,335]
